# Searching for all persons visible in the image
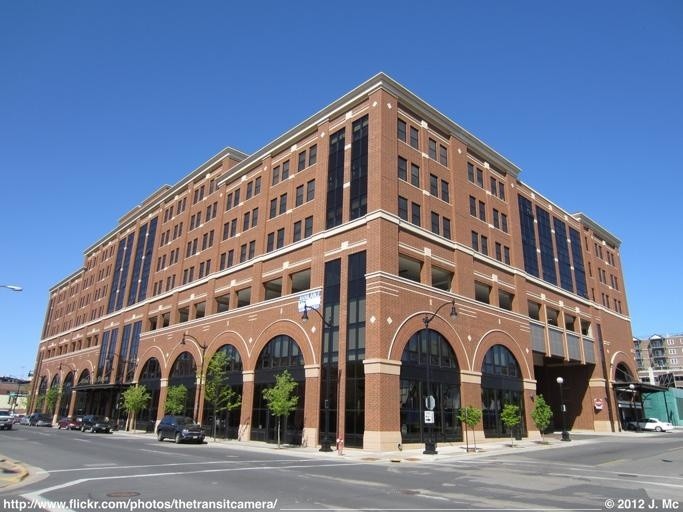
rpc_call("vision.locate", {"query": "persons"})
[336,437,344,457]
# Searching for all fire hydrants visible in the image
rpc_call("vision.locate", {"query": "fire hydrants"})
[334,433,344,456]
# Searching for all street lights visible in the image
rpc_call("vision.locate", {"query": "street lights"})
[179,331,208,424]
[0,284,23,292]
[422,297,458,455]
[105,350,126,430]
[26,369,44,414]
[556,376,571,442]
[628,383,642,432]
[301,302,334,454]
[58,362,75,418]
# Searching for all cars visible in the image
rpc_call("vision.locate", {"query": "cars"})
[12,414,29,426]
[57,415,80,430]
[624,416,674,432]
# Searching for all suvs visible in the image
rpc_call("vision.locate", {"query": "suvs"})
[155,415,205,445]
[0,410,12,430]
[29,414,52,426]
[80,414,111,433]
